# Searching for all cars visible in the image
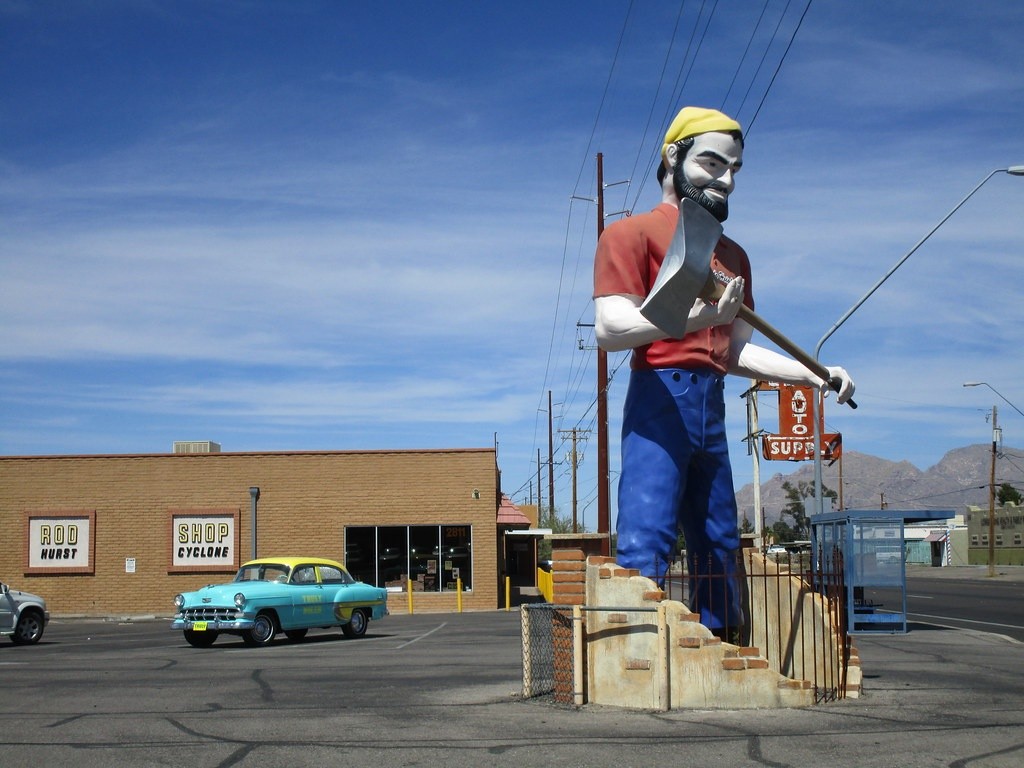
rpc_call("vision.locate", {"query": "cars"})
[1,579,52,648]
[347,542,471,575]
[169,556,389,649]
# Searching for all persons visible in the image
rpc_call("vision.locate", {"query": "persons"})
[593,106,854,647]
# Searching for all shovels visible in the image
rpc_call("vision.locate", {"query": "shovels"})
[632,192,862,411]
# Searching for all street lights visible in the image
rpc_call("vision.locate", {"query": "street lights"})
[961,380,1024,418]
[811,165,1024,600]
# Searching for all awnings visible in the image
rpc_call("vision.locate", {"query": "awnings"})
[923,533,947,542]
